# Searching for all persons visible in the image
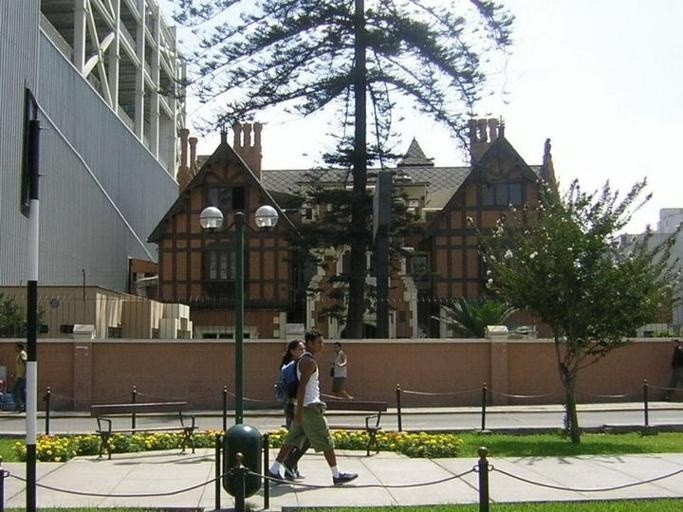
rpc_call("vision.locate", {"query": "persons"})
[662,339,683,401]
[328,342,355,401]
[279,338,306,480]
[267,328,358,485]
[10,342,28,412]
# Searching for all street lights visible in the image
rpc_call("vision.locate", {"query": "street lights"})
[201,205,277,423]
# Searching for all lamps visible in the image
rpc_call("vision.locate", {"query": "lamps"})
[322,401,387,457]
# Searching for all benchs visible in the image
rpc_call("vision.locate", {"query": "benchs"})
[91,402,199,459]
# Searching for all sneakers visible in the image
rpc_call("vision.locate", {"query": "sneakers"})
[333,473,358,484]
[282,460,295,478]
[284,471,304,479]
[268,469,283,481]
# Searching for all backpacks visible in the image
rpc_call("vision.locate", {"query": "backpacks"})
[279,353,315,397]
